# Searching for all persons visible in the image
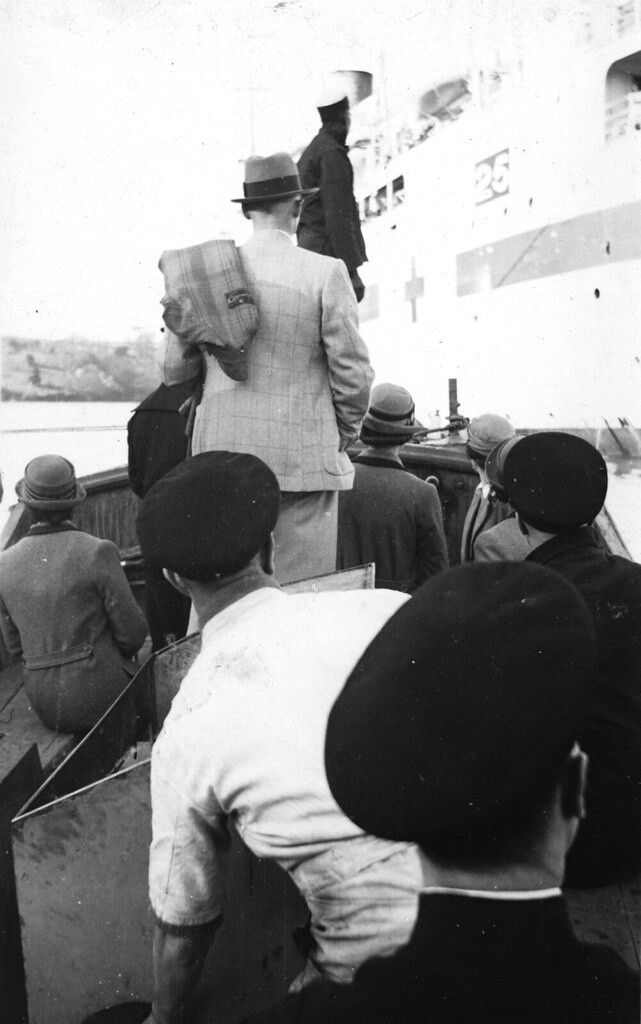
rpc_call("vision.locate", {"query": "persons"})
[128,374,202,653]
[143,450,422,1024]
[156,154,374,637]
[0,454,149,733]
[295,90,368,304]
[500,431,641,888]
[473,434,538,563]
[336,382,447,594]
[449,413,517,567]
[264,559,641,1023]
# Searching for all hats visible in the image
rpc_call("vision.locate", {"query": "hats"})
[231,151,321,203]
[363,383,416,435]
[503,431,608,531]
[316,94,350,120]
[137,450,281,580]
[15,455,87,509]
[485,433,528,488]
[323,559,601,839]
[466,413,514,454]
